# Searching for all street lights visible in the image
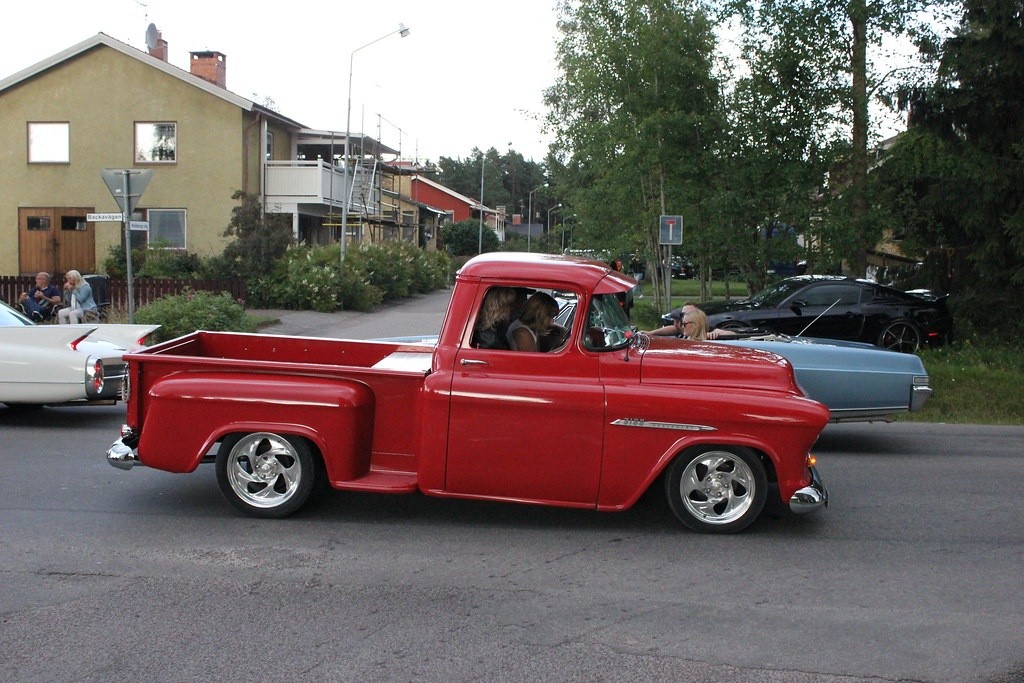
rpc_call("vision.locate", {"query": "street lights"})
[479,141,512,255]
[547,203,562,244]
[341,22,411,272]
[528,183,550,253]
[571,222,582,250]
[562,213,577,253]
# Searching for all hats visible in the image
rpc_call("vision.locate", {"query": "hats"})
[514,287,537,295]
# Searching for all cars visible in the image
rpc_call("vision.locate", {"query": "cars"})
[373,295,936,432]
[0,298,163,408]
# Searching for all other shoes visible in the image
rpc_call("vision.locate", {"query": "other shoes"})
[32,311,43,322]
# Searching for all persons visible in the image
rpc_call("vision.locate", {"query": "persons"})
[57,269,97,324]
[637,302,736,340]
[18,272,60,321]
[464,286,559,352]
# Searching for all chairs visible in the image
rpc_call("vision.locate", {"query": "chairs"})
[21,274,112,321]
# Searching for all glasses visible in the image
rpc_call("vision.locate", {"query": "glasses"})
[679,321,694,327]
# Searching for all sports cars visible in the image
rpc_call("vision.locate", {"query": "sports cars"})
[663,275,953,356]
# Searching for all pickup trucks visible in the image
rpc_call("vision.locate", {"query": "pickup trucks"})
[102,256,831,532]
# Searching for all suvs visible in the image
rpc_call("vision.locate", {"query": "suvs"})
[553,249,634,316]
